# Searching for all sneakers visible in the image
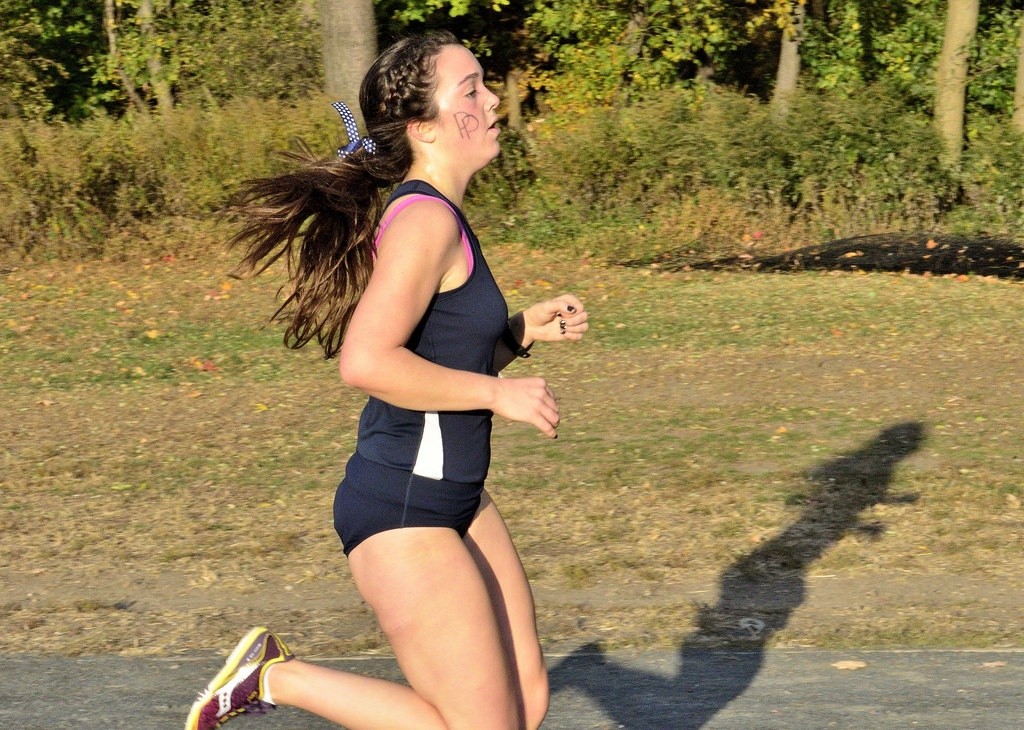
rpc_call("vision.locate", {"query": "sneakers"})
[182,626,295,730]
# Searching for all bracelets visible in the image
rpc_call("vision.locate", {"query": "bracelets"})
[502,319,535,358]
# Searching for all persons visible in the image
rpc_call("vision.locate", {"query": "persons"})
[182,30,588,728]
